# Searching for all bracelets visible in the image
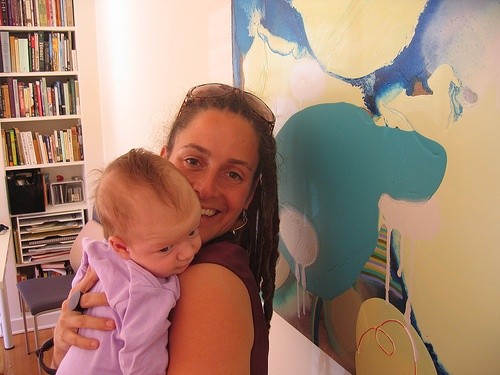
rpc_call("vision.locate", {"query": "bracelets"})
[34,335,57,375]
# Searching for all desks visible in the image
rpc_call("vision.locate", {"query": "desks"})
[0,225,14,350]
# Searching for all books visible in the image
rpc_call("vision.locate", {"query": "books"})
[0,30,76,72]
[0,77,80,118]
[17,262,75,283]
[52,184,83,204]
[1,124,83,167]
[0,0,75,27]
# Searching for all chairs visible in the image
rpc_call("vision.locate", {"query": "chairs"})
[16,204,98,375]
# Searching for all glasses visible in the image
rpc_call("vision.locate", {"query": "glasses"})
[178,83,276,137]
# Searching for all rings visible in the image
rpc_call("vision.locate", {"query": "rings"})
[68,290,89,312]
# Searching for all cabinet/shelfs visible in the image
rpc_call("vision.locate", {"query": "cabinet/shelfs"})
[0,0,103,335]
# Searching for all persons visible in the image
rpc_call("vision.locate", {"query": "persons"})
[48,147,202,375]
[50,84,282,375]
